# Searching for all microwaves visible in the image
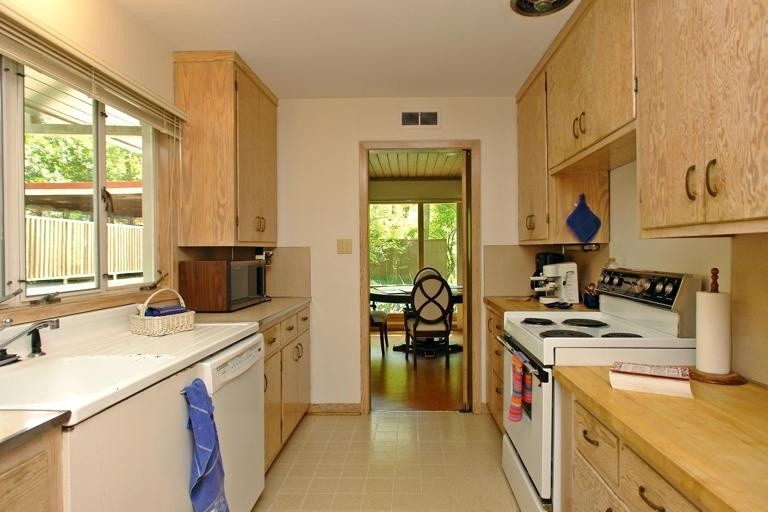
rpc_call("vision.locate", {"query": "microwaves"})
[180,258,269,310]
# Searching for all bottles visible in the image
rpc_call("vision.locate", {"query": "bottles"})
[604,257,619,270]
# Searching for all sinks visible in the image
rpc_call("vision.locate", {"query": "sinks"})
[0,352,176,406]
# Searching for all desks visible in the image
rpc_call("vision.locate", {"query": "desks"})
[370,285,463,360]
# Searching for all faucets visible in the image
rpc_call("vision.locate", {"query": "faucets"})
[0,318,60,350]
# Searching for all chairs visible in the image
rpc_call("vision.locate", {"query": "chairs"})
[405,276,453,370]
[404,267,441,319]
[370,301,389,358]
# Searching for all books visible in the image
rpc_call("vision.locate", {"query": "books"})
[607,362,694,400]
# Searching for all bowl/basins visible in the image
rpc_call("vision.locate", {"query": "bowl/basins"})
[581,293,599,309]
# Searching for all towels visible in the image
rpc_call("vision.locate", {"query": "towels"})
[183,378,229,512]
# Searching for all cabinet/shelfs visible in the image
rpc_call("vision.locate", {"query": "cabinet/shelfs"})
[194,297,311,474]
[546,0,635,176]
[637,0,768,239]
[551,365,768,512]
[173,51,279,250]
[483,296,600,435]
[516,71,610,246]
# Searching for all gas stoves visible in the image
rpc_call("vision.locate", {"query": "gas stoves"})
[505,312,674,343]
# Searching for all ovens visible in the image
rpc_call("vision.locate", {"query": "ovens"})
[496,331,553,500]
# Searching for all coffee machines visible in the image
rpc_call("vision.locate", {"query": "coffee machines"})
[531,253,566,299]
[539,261,578,304]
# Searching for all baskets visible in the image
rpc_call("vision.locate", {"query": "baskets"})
[131,288,194,336]
